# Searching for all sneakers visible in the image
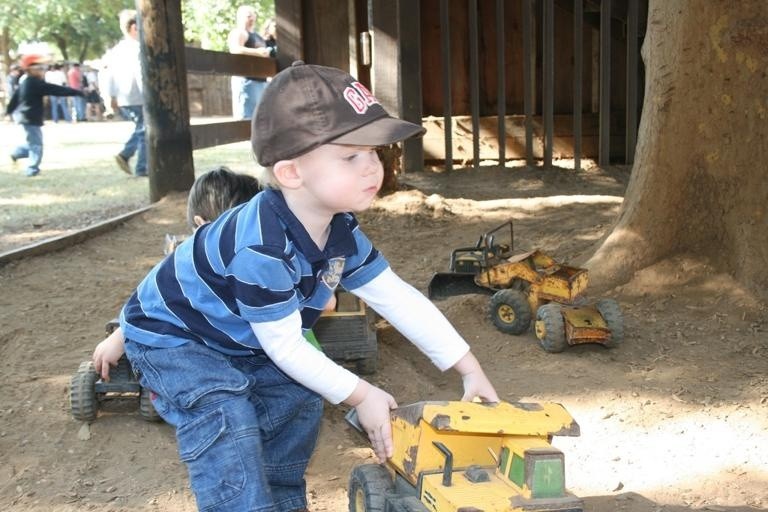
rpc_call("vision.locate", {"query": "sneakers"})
[115,153,133,175]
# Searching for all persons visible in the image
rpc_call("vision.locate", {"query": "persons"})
[91,168,268,380]
[2,55,94,178]
[6,60,107,122]
[259,17,277,56]
[116,59,499,512]
[227,5,268,122]
[98,8,149,179]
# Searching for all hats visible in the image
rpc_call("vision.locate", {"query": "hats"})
[250,61,427,169]
[20,51,53,68]
